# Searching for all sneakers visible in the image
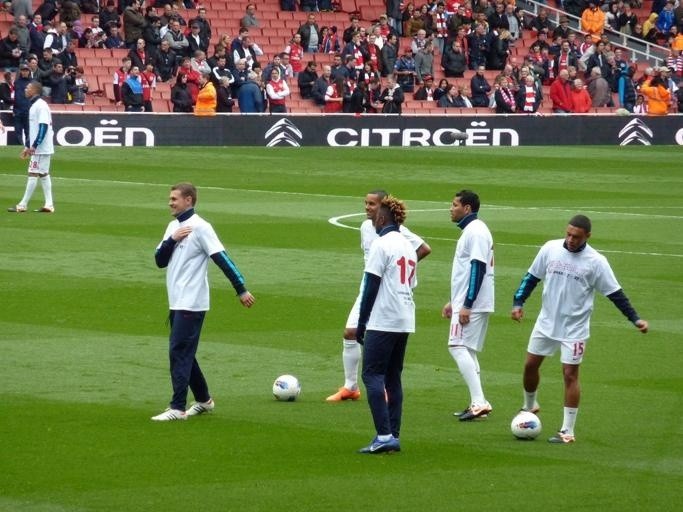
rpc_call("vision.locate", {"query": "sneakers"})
[547,432,575,442]
[325,387,360,401]
[359,436,396,454]
[185,399,214,415]
[32,206,54,213]
[7,205,26,212]
[459,400,491,420]
[453,406,487,417]
[150,407,187,421]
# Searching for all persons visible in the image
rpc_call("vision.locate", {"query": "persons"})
[325,190,433,404]
[7,80,55,213]
[149,181,256,423]
[1,1,683,116]
[444,189,497,423]
[510,213,648,447]
[13,66,33,147]
[354,194,418,455]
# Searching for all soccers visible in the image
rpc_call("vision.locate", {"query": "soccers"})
[272,375,300,401]
[511,412,541,439]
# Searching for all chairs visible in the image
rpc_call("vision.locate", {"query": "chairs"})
[0,2,681,116]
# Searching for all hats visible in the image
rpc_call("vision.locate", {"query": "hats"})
[20,64,30,71]
[379,14,387,20]
[404,46,412,52]
[659,66,670,72]
[559,17,569,24]
[425,75,433,81]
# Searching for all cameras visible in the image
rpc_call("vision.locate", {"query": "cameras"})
[68,65,77,75]
